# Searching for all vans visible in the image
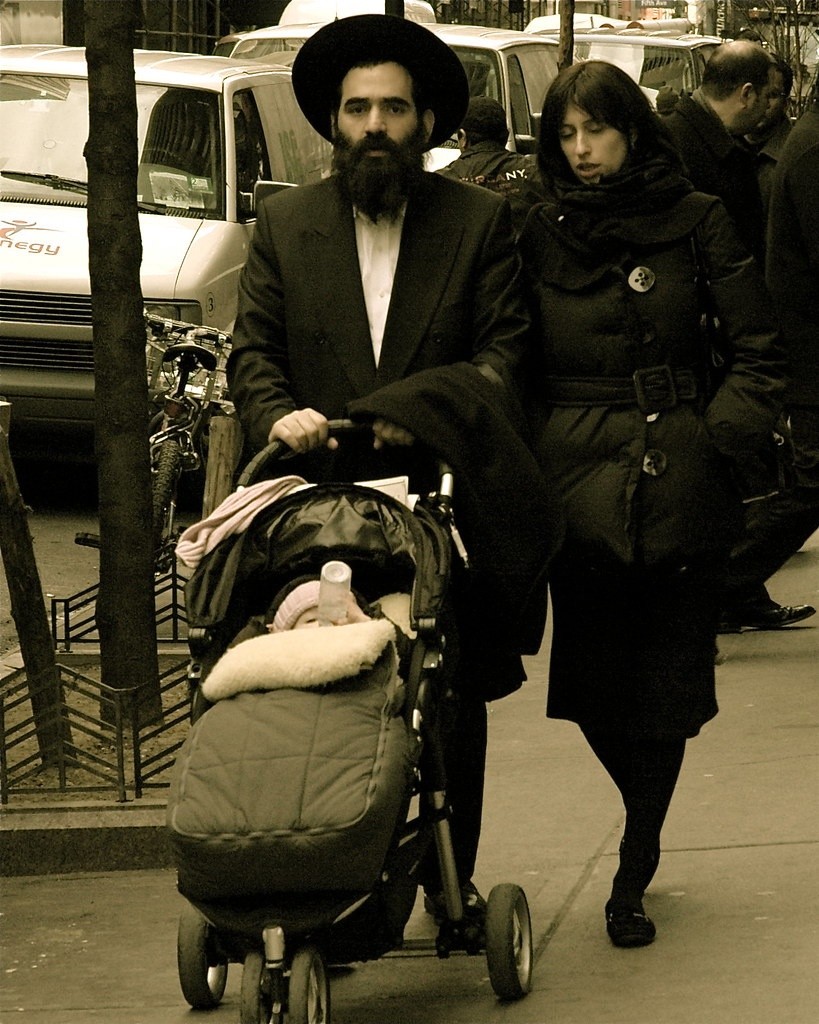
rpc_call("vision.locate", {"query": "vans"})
[207,1,734,177]
[0,42,338,516]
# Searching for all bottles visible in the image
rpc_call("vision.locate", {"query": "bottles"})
[317,560,351,628]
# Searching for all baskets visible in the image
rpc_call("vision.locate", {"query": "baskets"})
[145,326,235,407]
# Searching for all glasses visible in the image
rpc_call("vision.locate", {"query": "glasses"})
[768,87,786,98]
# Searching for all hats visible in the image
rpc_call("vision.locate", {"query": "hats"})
[291,14,470,152]
[461,95,506,133]
[656,85,679,113]
[266,574,358,634]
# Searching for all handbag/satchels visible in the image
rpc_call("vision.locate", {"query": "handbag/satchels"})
[774,436,819,503]
[414,493,527,702]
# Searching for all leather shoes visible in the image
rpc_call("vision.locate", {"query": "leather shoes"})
[605,900,656,942]
[719,590,816,631]
[619,838,625,852]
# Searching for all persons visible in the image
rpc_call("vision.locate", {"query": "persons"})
[261,570,384,632]
[225,15,819,946]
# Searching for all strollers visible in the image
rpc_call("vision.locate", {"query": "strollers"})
[165,415,534,1023]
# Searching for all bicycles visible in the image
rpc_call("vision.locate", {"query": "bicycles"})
[74,305,234,566]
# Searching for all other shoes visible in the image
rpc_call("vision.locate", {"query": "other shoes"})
[424,880,487,925]
